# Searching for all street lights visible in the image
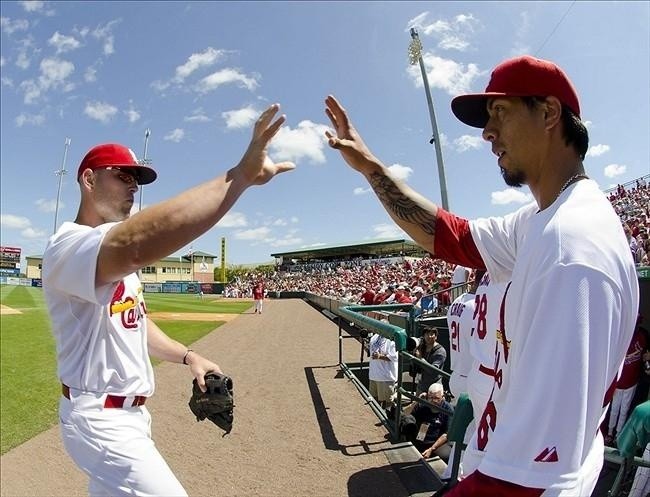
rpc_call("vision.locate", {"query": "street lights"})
[408,26,449,213]
[137,129,153,211]
[52,139,71,234]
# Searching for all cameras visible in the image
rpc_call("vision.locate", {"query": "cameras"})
[359,327,369,339]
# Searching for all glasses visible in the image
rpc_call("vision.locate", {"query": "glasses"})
[106,168,139,185]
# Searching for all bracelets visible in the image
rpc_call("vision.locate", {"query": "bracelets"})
[183,348,194,365]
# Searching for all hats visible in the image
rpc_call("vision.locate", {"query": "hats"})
[451,55,580,128]
[77,143,157,185]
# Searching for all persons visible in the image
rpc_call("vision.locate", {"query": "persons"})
[408,324,448,396]
[196,290,200,300]
[221,252,488,318]
[440,268,487,481]
[460,270,496,479]
[402,380,455,459]
[39,101,299,496]
[199,290,204,299]
[324,54,642,496]
[606,174,649,495]
[359,317,398,408]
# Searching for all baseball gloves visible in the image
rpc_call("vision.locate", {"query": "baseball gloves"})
[189,371,234,433]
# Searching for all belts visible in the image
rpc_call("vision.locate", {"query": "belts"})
[62,384,147,408]
